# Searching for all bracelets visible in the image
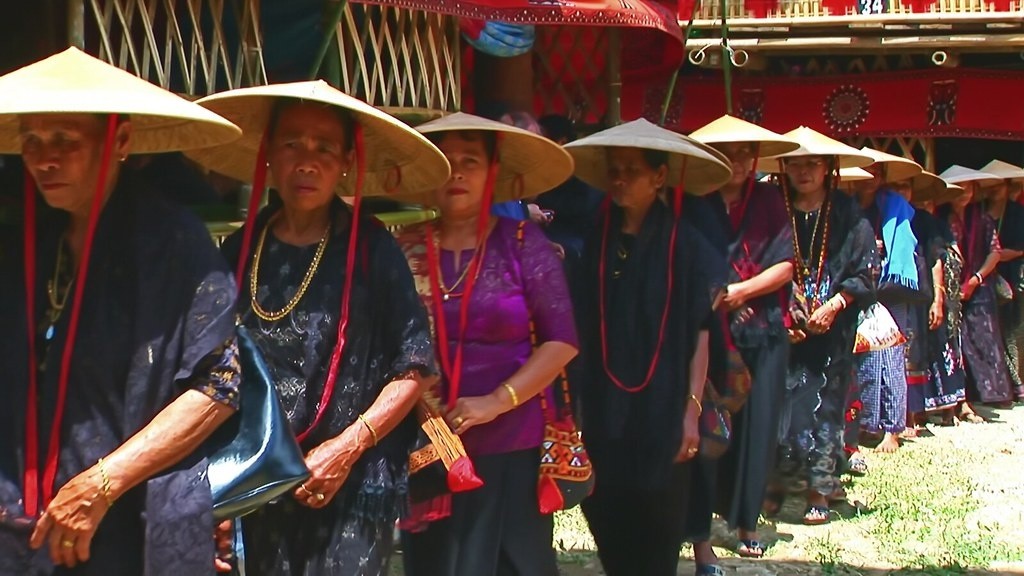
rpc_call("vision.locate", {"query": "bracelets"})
[98,457,116,506]
[357,415,379,447]
[500,382,519,408]
[686,394,703,416]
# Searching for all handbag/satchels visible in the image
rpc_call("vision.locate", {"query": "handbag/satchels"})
[536,413,597,515]
[698,375,733,461]
[852,299,909,355]
[207,324,315,524]
[719,347,753,416]
[994,268,1014,308]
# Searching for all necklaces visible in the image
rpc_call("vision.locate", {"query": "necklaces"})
[429,219,489,303]
[248,210,332,322]
[610,237,630,280]
[40,215,80,348]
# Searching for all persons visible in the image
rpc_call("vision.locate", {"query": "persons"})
[1,78,244,576]
[685,113,1024,575]
[548,124,713,576]
[207,82,438,574]
[384,117,581,574]
[484,109,566,262]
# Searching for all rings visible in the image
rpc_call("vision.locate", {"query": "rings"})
[454,417,463,424]
[60,540,74,550]
[316,493,325,503]
[301,485,313,495]
[688,447,698,454]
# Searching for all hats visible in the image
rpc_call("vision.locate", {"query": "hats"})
[0,45,1024,210]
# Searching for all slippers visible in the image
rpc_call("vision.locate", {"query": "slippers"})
[692,372,1023,576]
